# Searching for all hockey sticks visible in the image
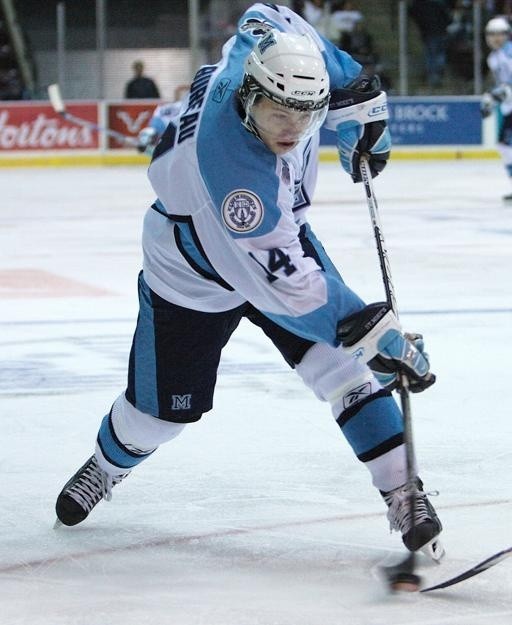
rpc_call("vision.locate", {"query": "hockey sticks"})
[360,155,416,583]
[48,83,137,144]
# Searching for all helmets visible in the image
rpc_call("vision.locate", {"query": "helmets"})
[485,18,509,34]
[236,27,332,144]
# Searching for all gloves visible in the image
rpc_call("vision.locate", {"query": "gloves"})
[478,86,506,117]
[335,300,437,394]
[326,75,393,184]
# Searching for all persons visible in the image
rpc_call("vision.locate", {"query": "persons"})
[55,0,444,556]
[120,55,160,102]
[412,0,511,96]
[302,0,390,94]
[477,14,511,201]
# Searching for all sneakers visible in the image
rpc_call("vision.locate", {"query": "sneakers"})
[379,472,444,555]
[54,451,126,527]
